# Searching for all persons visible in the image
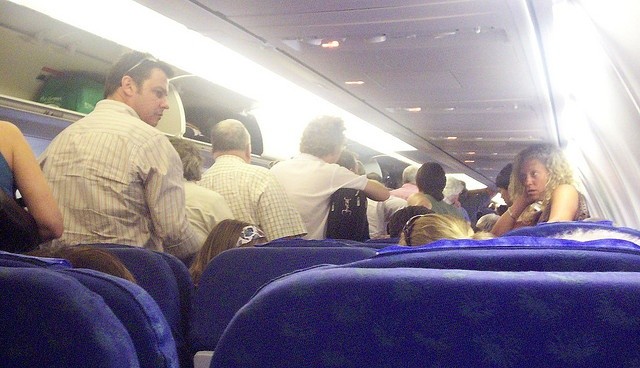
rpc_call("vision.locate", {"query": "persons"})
[1,120,65,253]
[400,213,491,246]
[492,143,594,237]
[61,248,135,284]
[187,219,268,284]
[365,171,407,240]
[492,162,515,216]
[443,175,471,225]
[458,183,500,226]
[475,212,500,232]
[168,136,233,249]
[337,149,364,177]
[197,118,306,241]
[391,160,423,203]
[35,50,200,265]
[400,162,466,217]
[270,115,392,241]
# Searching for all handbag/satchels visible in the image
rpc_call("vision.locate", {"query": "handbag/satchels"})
[0,186,40,253]
[328,188,366,243]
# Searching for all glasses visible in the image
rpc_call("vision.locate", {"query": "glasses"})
[235,225,266,248]
[402,213,424,245]
[120,56,158,86]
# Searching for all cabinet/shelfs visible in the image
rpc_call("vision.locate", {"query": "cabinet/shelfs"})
[0,0,281,170]
[337,137,411,191]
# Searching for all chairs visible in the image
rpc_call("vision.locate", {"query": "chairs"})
[0,217,640,367]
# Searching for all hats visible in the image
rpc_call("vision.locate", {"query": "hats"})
[496,163,512,189]
[441,177,464,196]
[403,163,423,184]
